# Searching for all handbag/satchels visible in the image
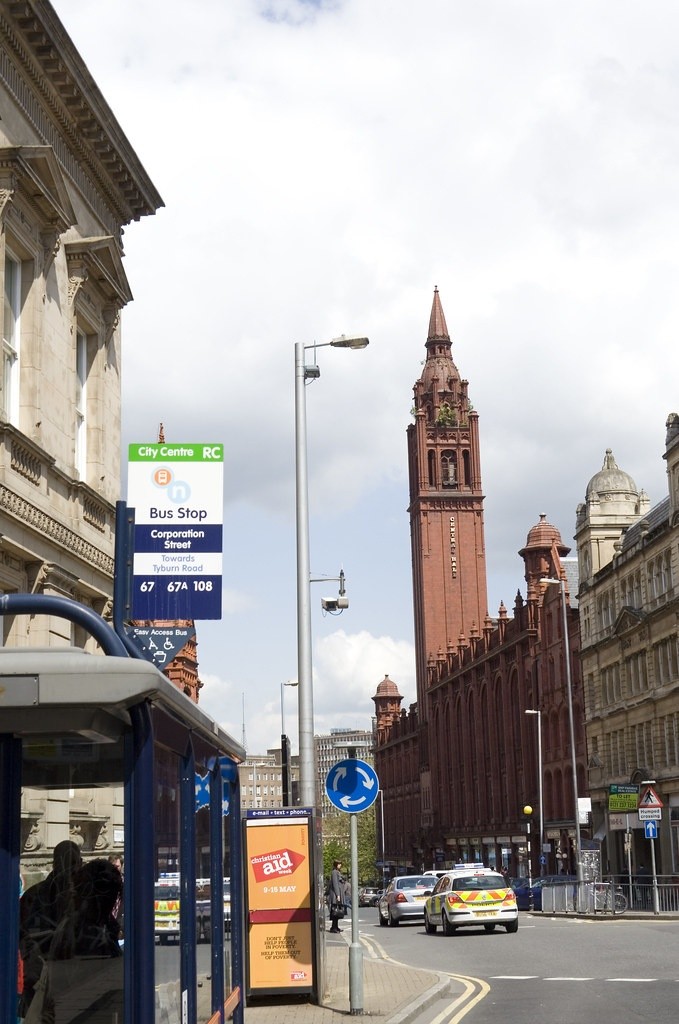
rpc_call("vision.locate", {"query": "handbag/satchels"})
[330,901,344,920]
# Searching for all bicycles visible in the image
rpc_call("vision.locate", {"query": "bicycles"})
[590,885,628,915]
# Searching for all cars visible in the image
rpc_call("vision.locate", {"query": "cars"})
[416,870,477,887]
[369,889,386,907]
[423,863,518,936]
[154,877,224,944]
[223,877,231,931]
[378,875,439,926]
[513,875,578,908]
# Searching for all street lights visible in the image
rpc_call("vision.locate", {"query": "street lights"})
[525,710,543,876]
[539,578,583,881]
[281,682,298,736]
[298,335,370,810]
[524,806,534,910]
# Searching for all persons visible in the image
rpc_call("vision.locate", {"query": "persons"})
[621,865,651,906]
[500,866,510,887]
[17,841,128,1024]
[325,860,352,933]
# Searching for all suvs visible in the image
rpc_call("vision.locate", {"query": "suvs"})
[358,888,379,907]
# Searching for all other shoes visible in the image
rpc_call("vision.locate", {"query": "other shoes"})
[336,927,343,932]
[330,928,337,933]
